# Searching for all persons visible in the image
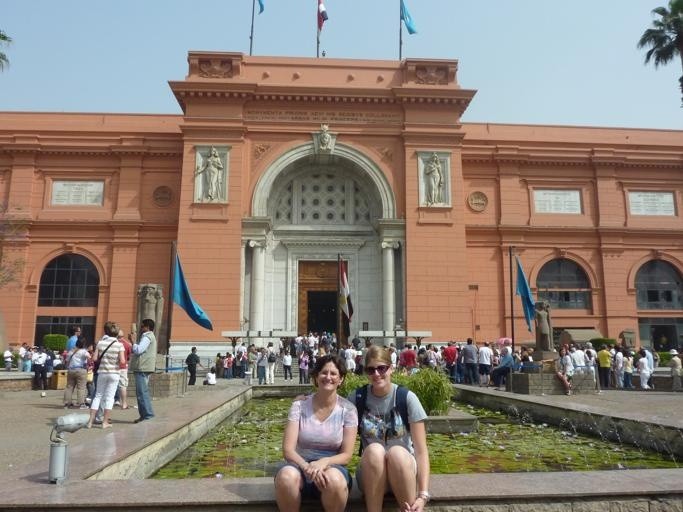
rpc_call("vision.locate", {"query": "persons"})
[84,343,97,369]
[669,349,683,391]
[185,347,216,385]
[4,342,65,390]
[535,302,551,351]
[128,319,158,423]
[90,321,113,424]
[557,342,659,395]
[425,154,443,204]
[66,324,80,353]
[342,345,431,511]
[216,342,275,384]
[280,331,368,384]
[115,329,132,410]
[197,147,223,200]
[385,338,533,389]
[62,336,90,410]
[83,320,126,429]
[272,355,361,511]
[134,284,164,331]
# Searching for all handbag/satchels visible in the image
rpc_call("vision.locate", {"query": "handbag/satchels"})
[93,358,100,373]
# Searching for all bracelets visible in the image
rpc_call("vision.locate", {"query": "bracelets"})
[417,490,430,503]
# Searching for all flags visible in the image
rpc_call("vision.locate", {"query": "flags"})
[257,0,265,14]
[400,1,418,34]
[514,255,535,332]
[172,251,213,330]
[338,253,354,319]
[317,0,329,32]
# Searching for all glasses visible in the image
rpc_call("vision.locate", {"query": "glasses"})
[364,365,389,375]
